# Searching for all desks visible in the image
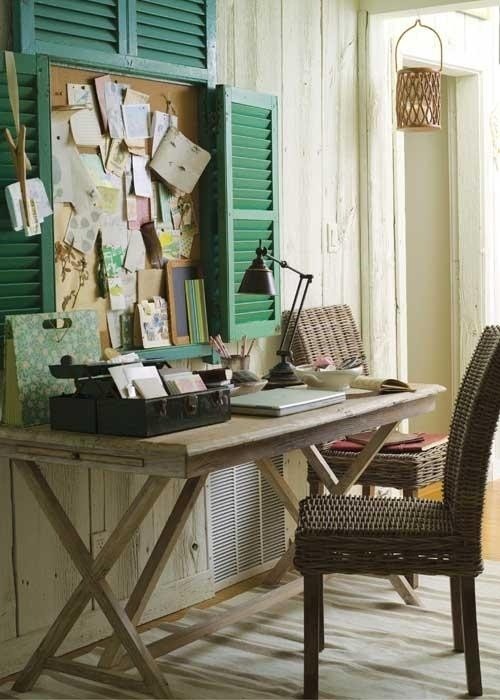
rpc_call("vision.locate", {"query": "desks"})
[0,367,452,700]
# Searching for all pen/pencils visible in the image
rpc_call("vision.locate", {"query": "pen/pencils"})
[209,334,255,360]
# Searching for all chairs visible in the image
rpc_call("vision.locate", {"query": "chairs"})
[292,321,498,699]
[282,303,452,593]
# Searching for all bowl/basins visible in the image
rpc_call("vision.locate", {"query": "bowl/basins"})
[293,364,363,391]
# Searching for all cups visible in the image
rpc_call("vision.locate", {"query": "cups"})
[221,355,249,370]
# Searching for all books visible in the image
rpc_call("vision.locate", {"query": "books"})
[330,433,451,453]
[108,361,211,404]
[334,375,417,399]
[147,125,213,199]
[344,430,424,448]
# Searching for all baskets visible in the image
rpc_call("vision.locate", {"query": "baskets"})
[396,66,441,132]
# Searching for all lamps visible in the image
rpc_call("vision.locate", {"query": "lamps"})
[238,237,314,392]
